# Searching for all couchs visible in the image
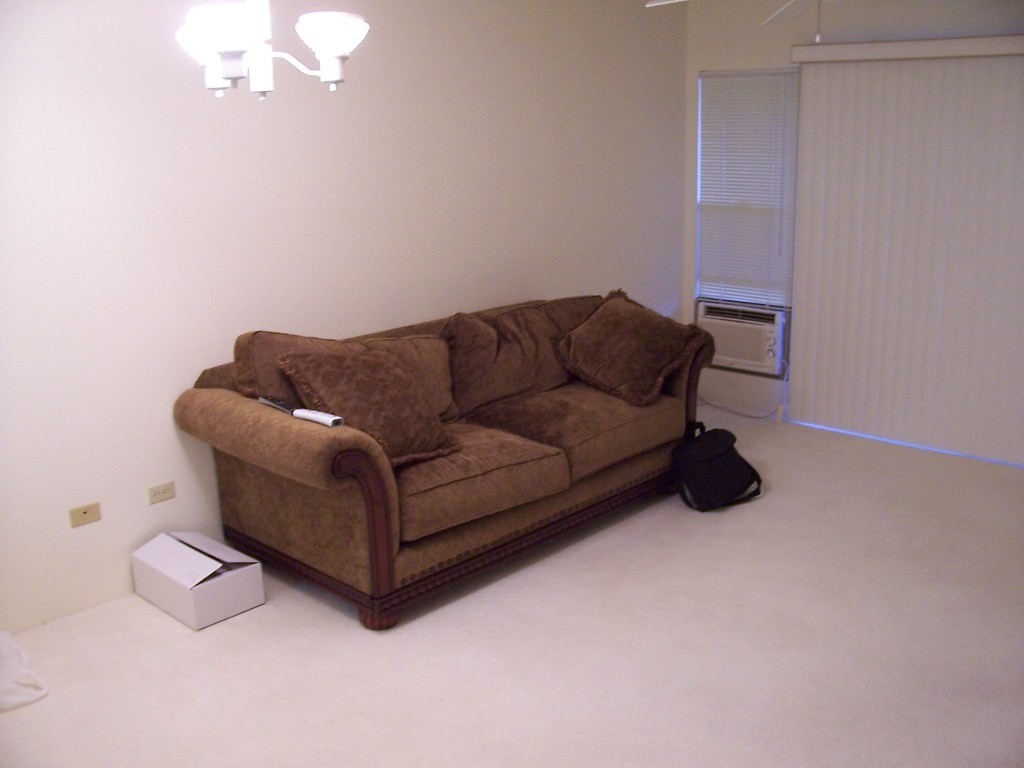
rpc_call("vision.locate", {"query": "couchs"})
[175,295,717,630]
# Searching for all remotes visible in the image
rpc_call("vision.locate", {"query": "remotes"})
[258,396,295,415]
[292,409,343,428]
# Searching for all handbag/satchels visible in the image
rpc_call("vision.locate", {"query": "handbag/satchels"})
[673,421,761,512]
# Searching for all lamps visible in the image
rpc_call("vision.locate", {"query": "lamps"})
[174,0,370,103]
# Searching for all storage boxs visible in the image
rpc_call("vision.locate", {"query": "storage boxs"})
[130,531,265,634]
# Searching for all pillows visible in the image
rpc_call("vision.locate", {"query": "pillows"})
[277,351,463,468]
[550,288,703,408]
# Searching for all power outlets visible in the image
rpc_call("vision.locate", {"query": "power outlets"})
[148,481,175,505]
